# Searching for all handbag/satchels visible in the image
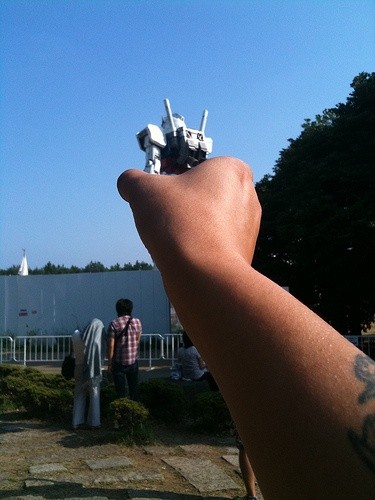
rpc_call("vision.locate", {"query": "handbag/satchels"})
[61,337,75,381]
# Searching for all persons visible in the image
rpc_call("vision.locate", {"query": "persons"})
[233,423,256,500]
[106,299,142,404]
[177,331,220,391]
[116,155,375,500]
[72,318,106,430]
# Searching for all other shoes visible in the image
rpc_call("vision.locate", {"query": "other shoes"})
[72,426,97,434]
